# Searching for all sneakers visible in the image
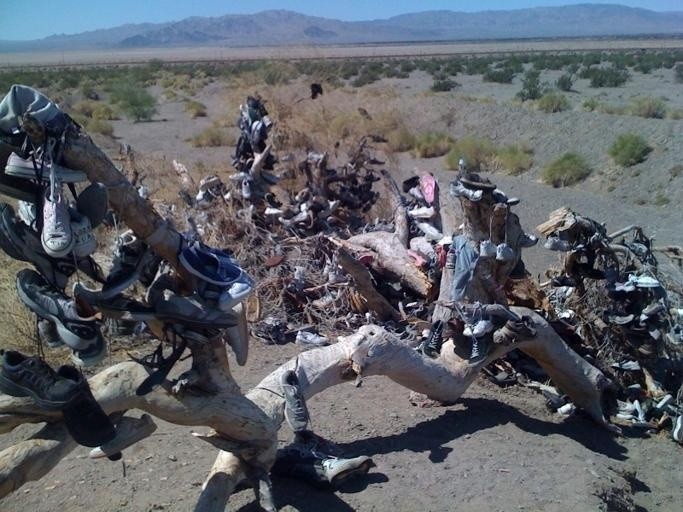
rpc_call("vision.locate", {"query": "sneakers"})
[482,363,517,385]
[250,314,329,346]
[534,263,683,356]
[542,389,577,419]
[0,129,255,366]
[279,370,308,434]
[88,413,158,460]
[54,363,117,447]
[194,175,227,210]
[278,432,373,489]
[0,349,80,407]
[224,96,395,240]
[545,235,574,252]
[402,159,539,369]
[269,264,366,314]
[610,359,641,371]
